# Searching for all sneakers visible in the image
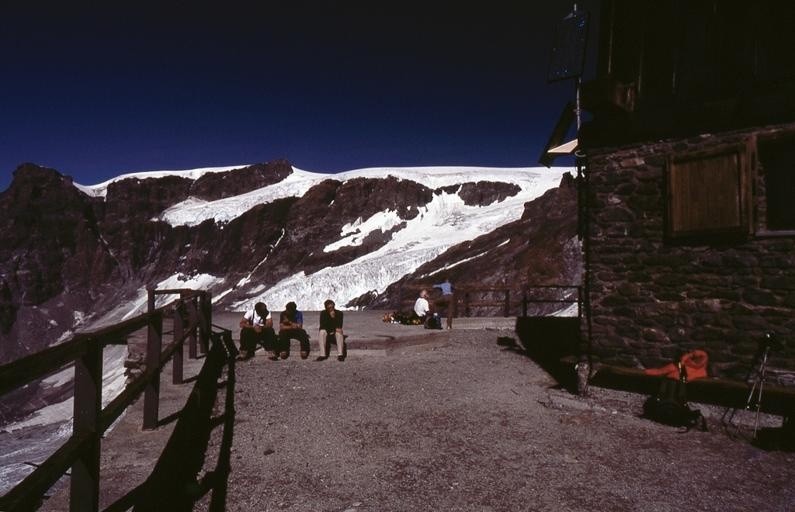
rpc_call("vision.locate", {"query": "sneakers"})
[338,356,344,361]
[317,356,327,361]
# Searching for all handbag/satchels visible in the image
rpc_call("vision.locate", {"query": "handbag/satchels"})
[424,315,441,329]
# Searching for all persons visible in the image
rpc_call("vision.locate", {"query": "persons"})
[413,289,432,325]
[432,278,464,330]
[237,302,280,361]
[316,300,345,361]
[278,302,310,359]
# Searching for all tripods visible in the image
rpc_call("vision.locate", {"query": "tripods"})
[721,333,774,439]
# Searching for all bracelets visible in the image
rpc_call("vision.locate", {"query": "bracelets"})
[287,320,290,323]
